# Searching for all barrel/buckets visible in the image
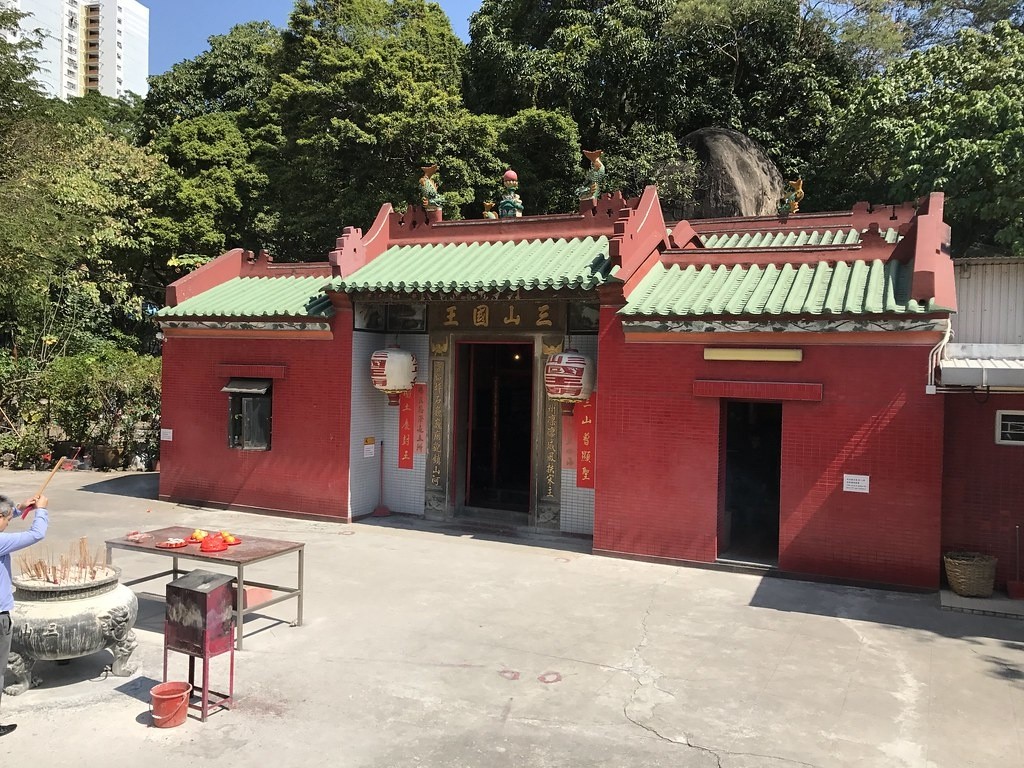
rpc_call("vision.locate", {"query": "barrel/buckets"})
[149,681,192,728]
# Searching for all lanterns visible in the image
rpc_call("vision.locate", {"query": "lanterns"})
[544,349,595,416]
[370,344,418,407]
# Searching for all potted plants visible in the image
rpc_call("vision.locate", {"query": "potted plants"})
[39,340,163,472]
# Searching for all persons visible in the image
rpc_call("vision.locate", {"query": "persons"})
[0,493,49,736]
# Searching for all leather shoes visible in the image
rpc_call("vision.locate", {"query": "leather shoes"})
[0,724,17,737]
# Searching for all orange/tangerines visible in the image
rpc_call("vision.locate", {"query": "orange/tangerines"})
[191,531,203,540]
[221,531,235,543]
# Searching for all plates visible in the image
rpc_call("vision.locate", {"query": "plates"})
[200,546,226,552]
[157,540,187,549]
[186,533,209,542]
[225,538,241,545]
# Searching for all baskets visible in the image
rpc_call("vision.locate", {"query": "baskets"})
[944,554,998,597]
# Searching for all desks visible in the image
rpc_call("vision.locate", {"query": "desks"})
[99,523,305,645]
